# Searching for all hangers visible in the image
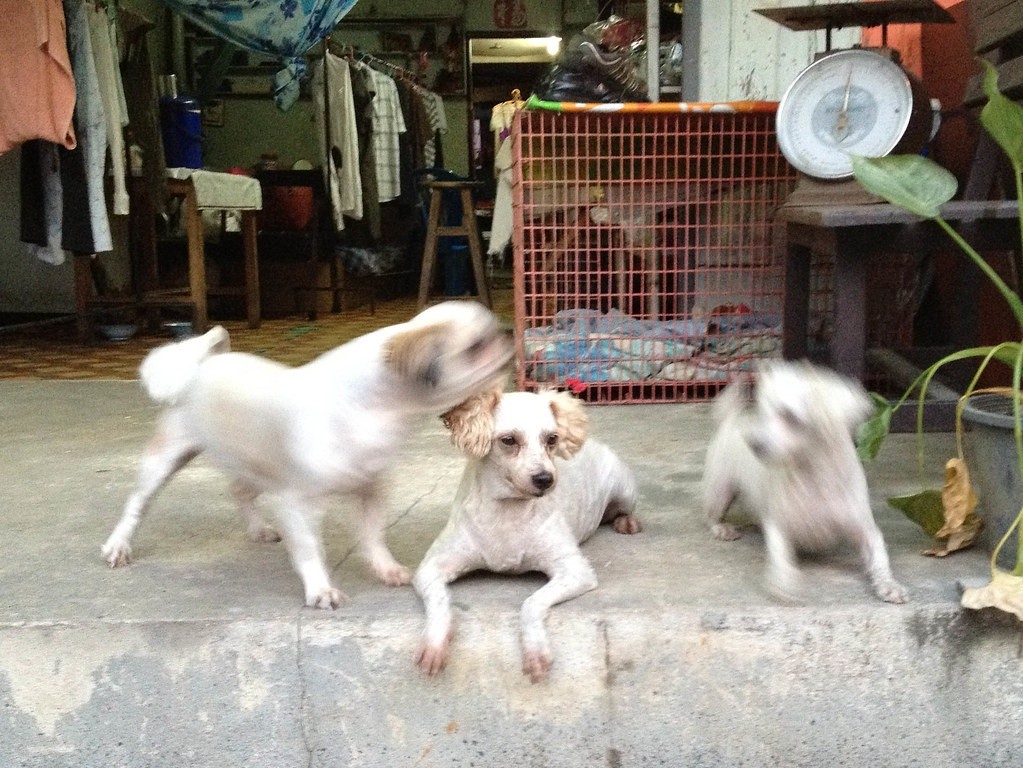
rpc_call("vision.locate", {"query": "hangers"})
[325,37,418,85]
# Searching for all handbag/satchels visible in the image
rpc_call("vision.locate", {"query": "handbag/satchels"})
[539,14,652,103]
[160,93,204,168]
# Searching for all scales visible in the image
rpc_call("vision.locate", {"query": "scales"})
[753,0,959,204]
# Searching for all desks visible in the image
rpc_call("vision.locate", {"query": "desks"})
[84,173,261,333]
[782,200,1023,432]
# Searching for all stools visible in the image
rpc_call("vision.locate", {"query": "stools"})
[417,181,491,311]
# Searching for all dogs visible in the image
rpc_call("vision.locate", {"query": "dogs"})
[699,360,910,609]
[97,298,642,685]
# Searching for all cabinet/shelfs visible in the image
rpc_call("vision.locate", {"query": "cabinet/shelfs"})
[337,13,469,96]
[170,8,311,104]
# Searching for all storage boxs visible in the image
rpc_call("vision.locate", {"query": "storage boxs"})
[264,290,335,313]
[515,108,928,405]
